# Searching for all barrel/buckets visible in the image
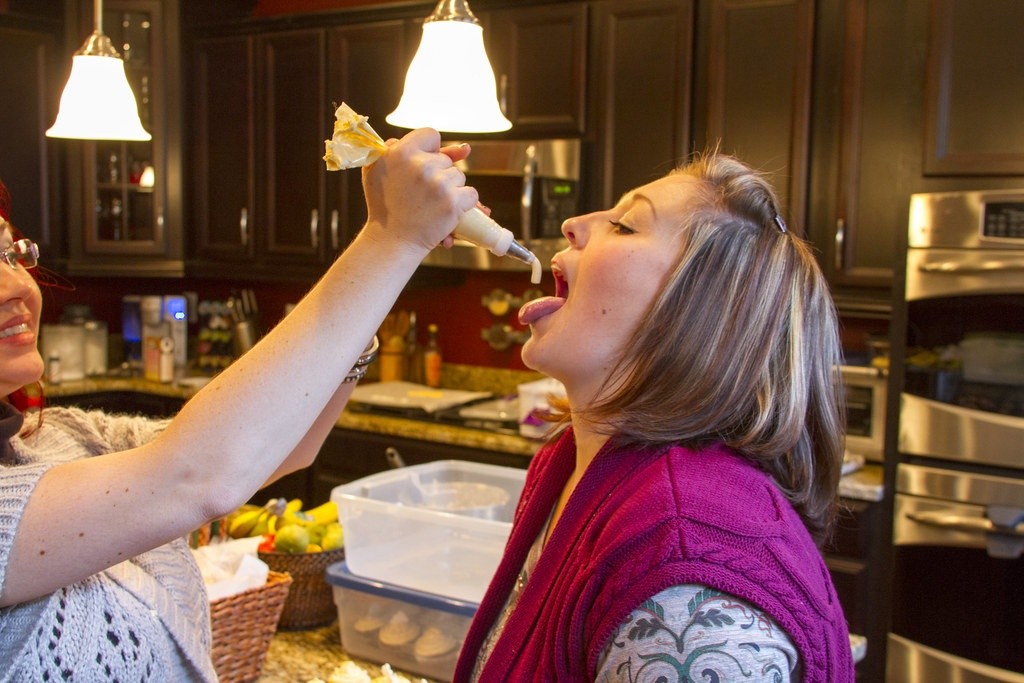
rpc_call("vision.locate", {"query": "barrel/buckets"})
[396,481,511,523]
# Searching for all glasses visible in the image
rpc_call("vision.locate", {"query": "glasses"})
[0,238,40,270]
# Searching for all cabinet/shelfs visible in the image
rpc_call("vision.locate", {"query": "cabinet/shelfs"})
[35,0,1024,683]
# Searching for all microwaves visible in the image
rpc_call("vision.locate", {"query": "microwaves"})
[414,124,580,272]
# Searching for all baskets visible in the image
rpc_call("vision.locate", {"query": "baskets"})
[258,549,345,630]
[209,570,293,683]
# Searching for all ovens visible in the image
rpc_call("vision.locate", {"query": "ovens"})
[885,188,1024,683]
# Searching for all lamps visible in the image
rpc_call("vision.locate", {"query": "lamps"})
[45,1,153,141]
[386,0,512,134]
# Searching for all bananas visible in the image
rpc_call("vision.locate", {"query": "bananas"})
[230,499,337,540]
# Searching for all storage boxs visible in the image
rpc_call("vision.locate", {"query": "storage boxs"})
[327,460,528,599]
[325,561,478,681]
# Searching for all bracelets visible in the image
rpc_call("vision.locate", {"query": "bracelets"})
[351,335,380,372]
[344,365,368,383]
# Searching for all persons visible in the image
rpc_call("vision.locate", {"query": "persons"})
[451,155,854,683]
[0,127,491,683]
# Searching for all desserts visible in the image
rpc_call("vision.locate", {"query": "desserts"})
[354,610,461,664]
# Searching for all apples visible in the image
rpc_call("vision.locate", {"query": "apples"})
[273,521,339,553]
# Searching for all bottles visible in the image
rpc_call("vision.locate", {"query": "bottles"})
[47,348,63,383]
[142,295,174,382]
[409,311,443,385]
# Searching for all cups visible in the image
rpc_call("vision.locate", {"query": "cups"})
[229,308,267,356]
[379,348,406,382]
[41,324,107,380]
